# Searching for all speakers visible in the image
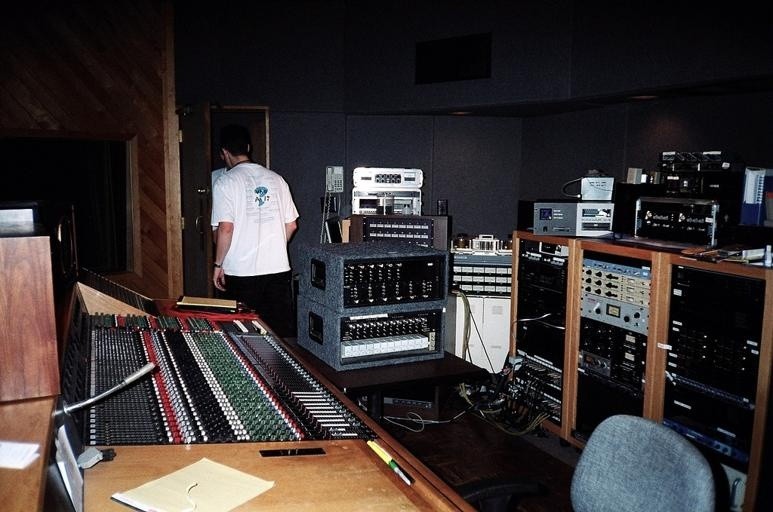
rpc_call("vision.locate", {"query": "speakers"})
[325,216,342,244]
[436,199,448,215]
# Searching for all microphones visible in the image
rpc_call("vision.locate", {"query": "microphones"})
[63,361,156,416]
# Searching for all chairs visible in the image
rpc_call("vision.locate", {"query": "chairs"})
[569,415,718,511]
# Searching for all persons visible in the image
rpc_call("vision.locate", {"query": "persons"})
[210,125,300,338]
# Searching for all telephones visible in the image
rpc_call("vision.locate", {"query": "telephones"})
[326,165,344,193]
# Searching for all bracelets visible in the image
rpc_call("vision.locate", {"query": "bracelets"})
[213,262,223,268]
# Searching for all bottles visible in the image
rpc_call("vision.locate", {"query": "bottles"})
[457,232,466,248]
[507,234,513,249]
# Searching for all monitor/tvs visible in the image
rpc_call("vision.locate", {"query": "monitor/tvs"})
[58,205,79,277]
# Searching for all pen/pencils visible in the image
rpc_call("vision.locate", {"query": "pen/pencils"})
[365,439,415,487]
